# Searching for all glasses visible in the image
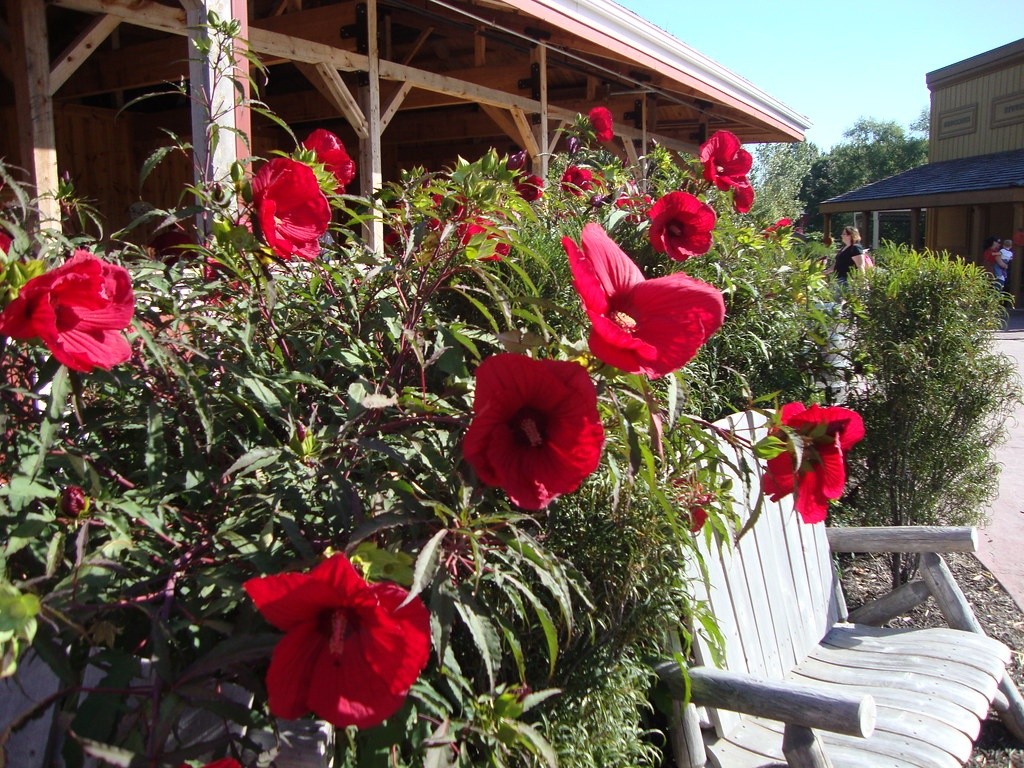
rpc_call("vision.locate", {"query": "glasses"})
[841,233,849,238]
[996,240,1001,245]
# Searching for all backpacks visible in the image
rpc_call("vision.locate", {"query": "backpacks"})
[849,243,874,269]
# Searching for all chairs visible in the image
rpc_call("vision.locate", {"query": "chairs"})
[643,407,1024,767]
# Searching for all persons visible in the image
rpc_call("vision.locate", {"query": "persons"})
[983,236,1013,298]
[824,225,866,287]
